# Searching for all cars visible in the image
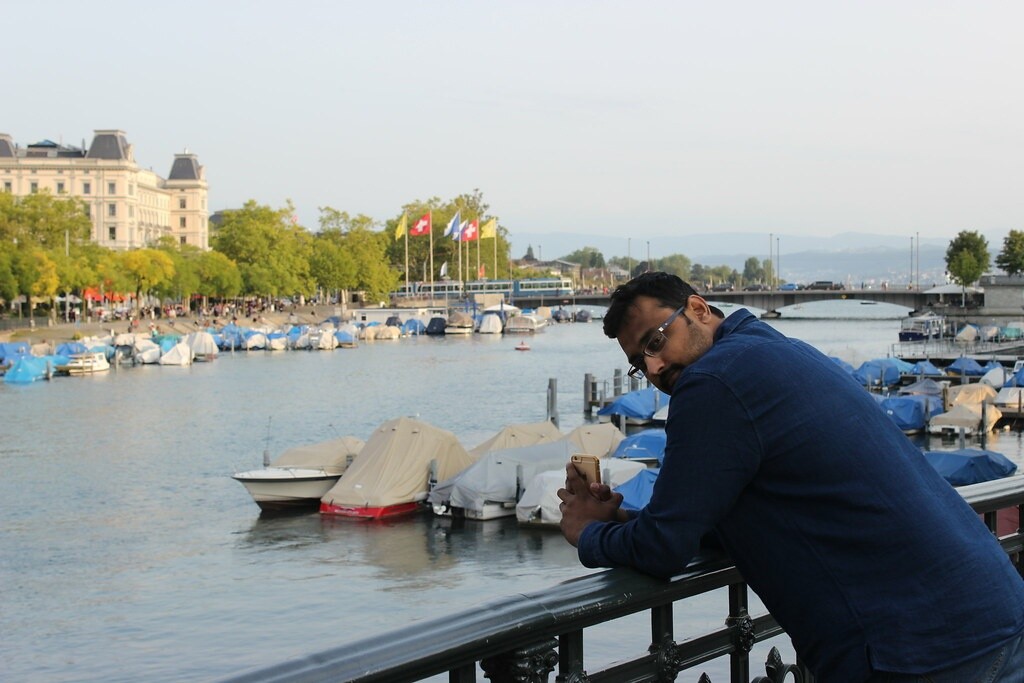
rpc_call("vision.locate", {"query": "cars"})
[743,284,765,292]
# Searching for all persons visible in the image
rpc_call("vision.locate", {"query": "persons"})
[557,270,1024,682]
[60,286,336,326]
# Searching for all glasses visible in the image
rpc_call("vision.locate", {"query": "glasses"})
[627,302,687,381]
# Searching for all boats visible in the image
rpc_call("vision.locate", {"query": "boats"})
[0,299,1024,530]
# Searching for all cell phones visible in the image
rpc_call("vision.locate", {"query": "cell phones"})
[571,454,602,501]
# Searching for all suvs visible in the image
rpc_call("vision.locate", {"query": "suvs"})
[777,283,799,291]
[712,284,734,292]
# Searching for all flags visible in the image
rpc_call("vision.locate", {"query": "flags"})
[393,208,498,243]
[440,261,447,277]
[479,263,485,276]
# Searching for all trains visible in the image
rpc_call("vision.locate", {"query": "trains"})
[388,275,575,298]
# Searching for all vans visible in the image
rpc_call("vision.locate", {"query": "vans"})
[807,281,833,291]
